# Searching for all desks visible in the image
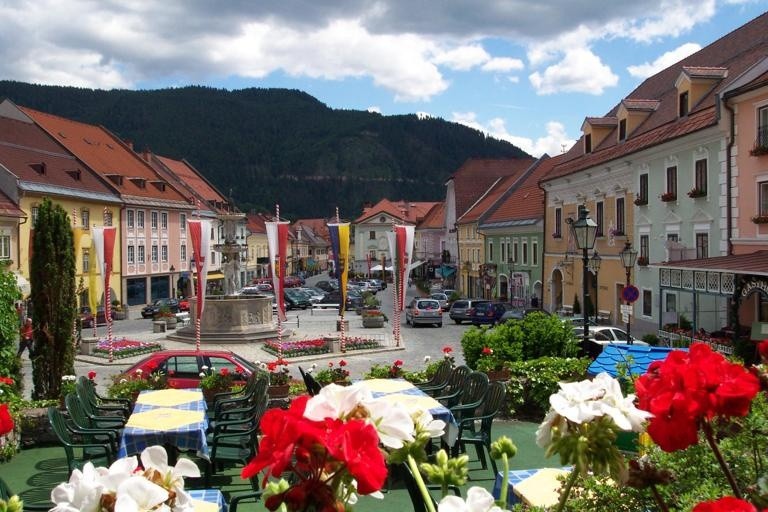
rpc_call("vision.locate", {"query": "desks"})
[492,467,574,512]
[125,388,207,460]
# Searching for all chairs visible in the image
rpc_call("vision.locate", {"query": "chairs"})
[48,376,131,471]
[414,358,507,478]
[211,372,271,494]
[298,366,322,395]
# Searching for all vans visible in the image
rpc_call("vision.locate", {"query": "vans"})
[449,297,492,325]
[429,288,456,311]
[403,296,442,327]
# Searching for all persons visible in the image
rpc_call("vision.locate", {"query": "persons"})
[17,317,35,360]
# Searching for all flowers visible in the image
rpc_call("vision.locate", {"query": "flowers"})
[121,370,174,386]
[255,359,292,377]
[438,485,502,512]
[390,360,404,372]
[307,361,349,377]
[62,371,96,383]
[200,365,244,380]
[241,393,448,512]
[47,445,222,512]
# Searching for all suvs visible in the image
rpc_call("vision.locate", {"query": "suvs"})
[469,302,515,327]
[499,307,551,323]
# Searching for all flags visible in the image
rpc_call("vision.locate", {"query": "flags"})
[393,224,415,310]
[186,218,212,321]
[87,240,96,318]
[326,222,350,317]
[71,226,87,261]
[103,227,117,324]
[265,221,289,322]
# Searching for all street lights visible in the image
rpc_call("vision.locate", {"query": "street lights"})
[168,264,176,301]
[617,238,639,347]
[571,199,601,354]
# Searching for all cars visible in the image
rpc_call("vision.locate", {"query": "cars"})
[179,296,192,310]
[557,316,599,328]
[568,326,649,348]
[114,349,304,401]
[74,305,119,327]
[141,298,177,320]
[230,274,386,310]
